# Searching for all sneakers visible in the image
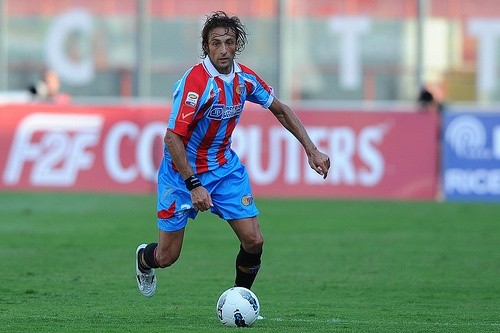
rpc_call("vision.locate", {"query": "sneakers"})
[136,244,156,298]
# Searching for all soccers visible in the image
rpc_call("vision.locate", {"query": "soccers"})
[216,287,260,327]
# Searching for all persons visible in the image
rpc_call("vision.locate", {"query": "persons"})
[136,9,330,321]
[36,69,70,104]
[420,90,449,112]
